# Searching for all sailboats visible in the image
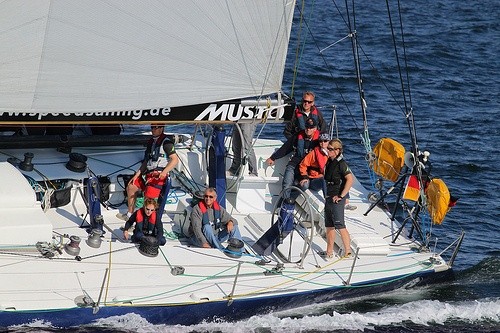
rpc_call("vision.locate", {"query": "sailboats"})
[0,2,466,332]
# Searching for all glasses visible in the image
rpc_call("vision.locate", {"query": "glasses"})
[146,208,154,211]
[151,126,161,129]
[327,147,340,151]
[205,195,213,198]
[305,125,315,129]
[319,140,327,142]
[303,100,313,104]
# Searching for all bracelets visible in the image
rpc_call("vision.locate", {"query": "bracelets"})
[229,220,233,223]
[338,195,343,199]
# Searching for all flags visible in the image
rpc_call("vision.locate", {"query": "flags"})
[403,176,421,201]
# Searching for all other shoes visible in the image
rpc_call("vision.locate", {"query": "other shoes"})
[340,252,353,259]
[317,250,334,259]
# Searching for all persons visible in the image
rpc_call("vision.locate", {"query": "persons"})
[315,140,353,259]
[116,123,178,231]
[123,198,166,246]
[267,118,322,200]
[226,122,259,177]
[299,134,357,210]
[188,187,244,250]
[284,92,327,142]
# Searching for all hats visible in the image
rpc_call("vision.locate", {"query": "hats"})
[305,118,317,126]
[318,133,331,141]
